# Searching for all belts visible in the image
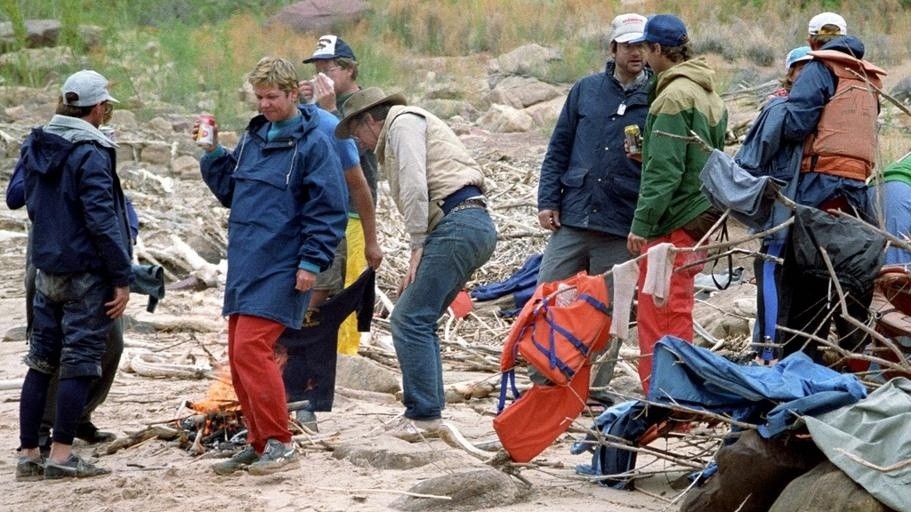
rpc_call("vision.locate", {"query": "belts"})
[447,198,487,214]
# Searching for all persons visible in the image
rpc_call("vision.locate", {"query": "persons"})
[866,150,911,272]
[527,12,656,403]
[623,14,728,436]
[14,69,134,482]
[333,86,498,442]
[191,56,350,476]
[5,93,138,450]
[766,45,811,104]
[297,33,378,354]
[764,10,888,371]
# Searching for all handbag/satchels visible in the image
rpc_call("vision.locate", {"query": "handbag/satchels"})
[683,204,733,291]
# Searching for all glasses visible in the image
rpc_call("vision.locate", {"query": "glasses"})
[311,66,339,76]
[618,42,647,54]
[104,104,114,114]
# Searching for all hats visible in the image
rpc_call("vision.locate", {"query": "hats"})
[62,69,121,108]
[807,12,848,37]
[629,14,690,47]
[334,85,409,141]
[785,46,813,77]
[610,12,648,45]
[301,34,356,63]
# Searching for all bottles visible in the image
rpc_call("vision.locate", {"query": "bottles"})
[195,114,215,149]
[626,125,643,156]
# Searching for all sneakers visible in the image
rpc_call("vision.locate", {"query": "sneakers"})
[15,454,47,482]
[42,454,112,479]
[247,437,302,476]
[211,443,262,476]
[385,416,445,443]
[74,420,116,443]
